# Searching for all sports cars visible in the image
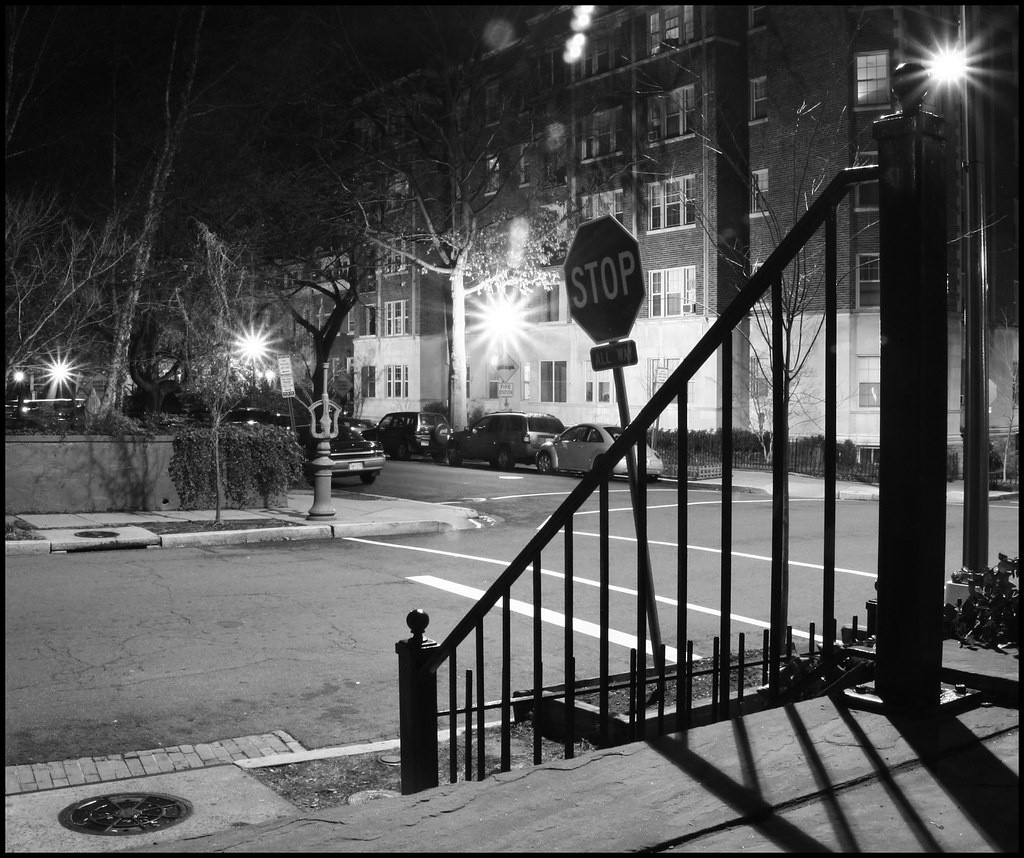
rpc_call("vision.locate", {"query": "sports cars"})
[536,423,663,484]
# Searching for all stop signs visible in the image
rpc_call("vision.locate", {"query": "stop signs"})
[565,213,647,344]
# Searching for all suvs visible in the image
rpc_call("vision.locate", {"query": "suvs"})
[359,410,454,462]
[446,411,565,472]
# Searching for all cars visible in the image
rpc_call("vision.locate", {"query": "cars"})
[113,388,377,447]
[289,423,387,487]
